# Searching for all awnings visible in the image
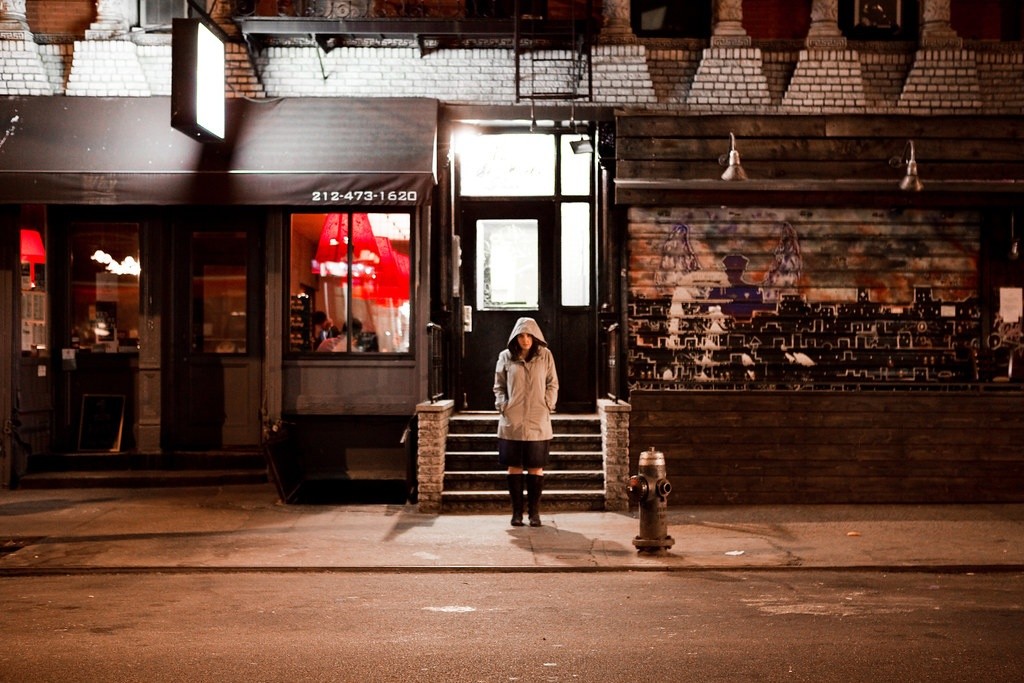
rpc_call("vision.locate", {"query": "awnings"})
[0,95,438,206]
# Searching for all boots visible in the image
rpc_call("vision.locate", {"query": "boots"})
[506,474,525,525]
[525,474,545,527]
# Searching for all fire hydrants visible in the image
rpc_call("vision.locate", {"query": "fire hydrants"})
[625,446,675,555]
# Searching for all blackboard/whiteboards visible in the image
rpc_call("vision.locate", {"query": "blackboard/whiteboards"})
[77,392,126,452]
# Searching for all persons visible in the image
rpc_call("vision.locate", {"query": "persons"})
[311,311,363,352]
[493,317,559,526]
